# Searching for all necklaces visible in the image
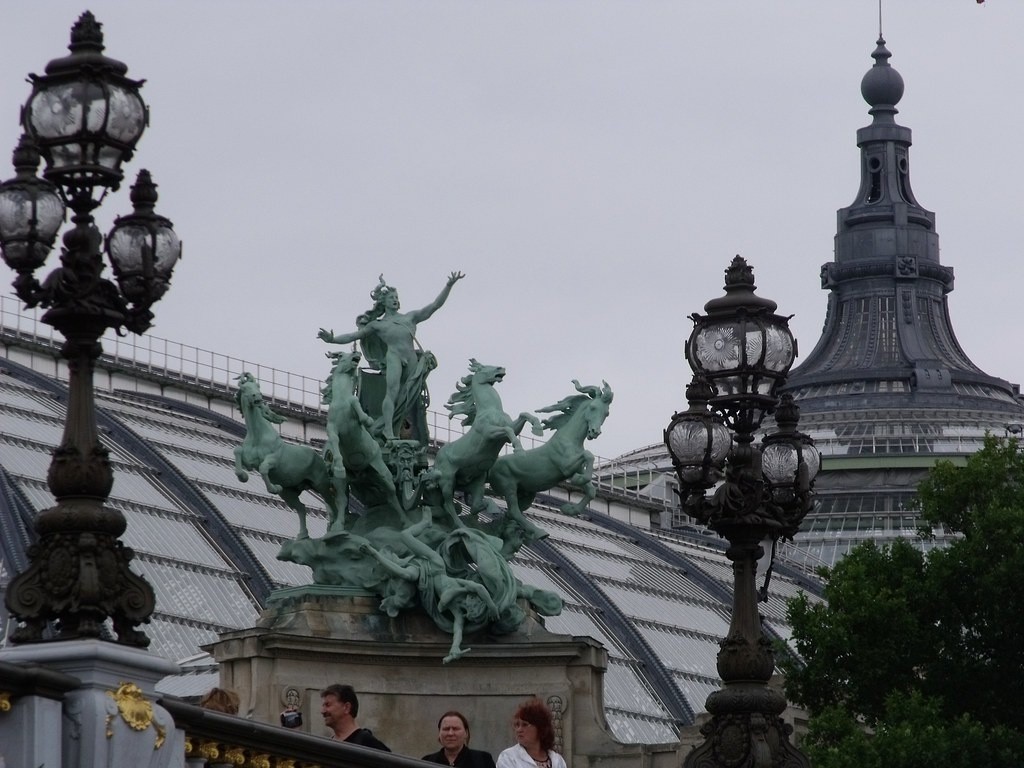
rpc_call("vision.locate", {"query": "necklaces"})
[532,756,552,768]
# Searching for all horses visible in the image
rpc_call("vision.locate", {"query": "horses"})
[486,379,614,563]
[319,351,415,532]
[422,358,544,529]
[233,371,338,542]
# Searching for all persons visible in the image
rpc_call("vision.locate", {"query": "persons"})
[316,271,467,439]
[321,685,391,752]
[496,699,567,768]
[421,711,496,768]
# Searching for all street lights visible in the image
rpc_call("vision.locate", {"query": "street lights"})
[661,256,825,768]
[0,10,199,766]
